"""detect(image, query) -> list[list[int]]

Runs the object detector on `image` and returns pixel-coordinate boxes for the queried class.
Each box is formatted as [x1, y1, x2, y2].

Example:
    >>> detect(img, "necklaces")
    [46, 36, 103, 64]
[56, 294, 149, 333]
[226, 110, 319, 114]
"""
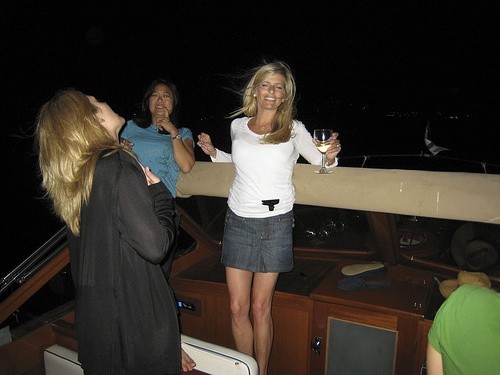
[252, 120, 273, 131]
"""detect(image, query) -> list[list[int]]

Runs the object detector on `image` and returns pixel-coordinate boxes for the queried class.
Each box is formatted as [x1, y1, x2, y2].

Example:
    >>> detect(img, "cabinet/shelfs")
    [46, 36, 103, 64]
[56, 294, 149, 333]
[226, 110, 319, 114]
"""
[168, 246, 449, 375]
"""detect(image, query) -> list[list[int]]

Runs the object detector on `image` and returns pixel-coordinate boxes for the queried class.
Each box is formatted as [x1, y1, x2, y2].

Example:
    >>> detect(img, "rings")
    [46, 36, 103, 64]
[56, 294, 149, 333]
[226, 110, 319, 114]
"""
[336, 145, 339, 147]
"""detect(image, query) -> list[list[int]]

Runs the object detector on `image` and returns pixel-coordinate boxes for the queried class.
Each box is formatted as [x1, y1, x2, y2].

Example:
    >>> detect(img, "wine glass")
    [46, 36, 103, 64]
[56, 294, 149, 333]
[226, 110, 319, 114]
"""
[314, 129, 333, 175]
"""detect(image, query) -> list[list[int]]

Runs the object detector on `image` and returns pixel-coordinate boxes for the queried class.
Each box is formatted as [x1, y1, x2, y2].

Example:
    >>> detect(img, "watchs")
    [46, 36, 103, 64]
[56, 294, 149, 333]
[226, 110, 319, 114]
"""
[172, 135, 181, 140]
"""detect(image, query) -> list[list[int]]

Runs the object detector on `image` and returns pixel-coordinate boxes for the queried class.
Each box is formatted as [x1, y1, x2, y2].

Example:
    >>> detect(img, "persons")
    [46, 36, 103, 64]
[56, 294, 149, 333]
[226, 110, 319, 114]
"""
[36, 90, 182, 375]
[426, 284, 500, 375]
[120, 79, 196, 372]
[197, 63, 341, 375]
